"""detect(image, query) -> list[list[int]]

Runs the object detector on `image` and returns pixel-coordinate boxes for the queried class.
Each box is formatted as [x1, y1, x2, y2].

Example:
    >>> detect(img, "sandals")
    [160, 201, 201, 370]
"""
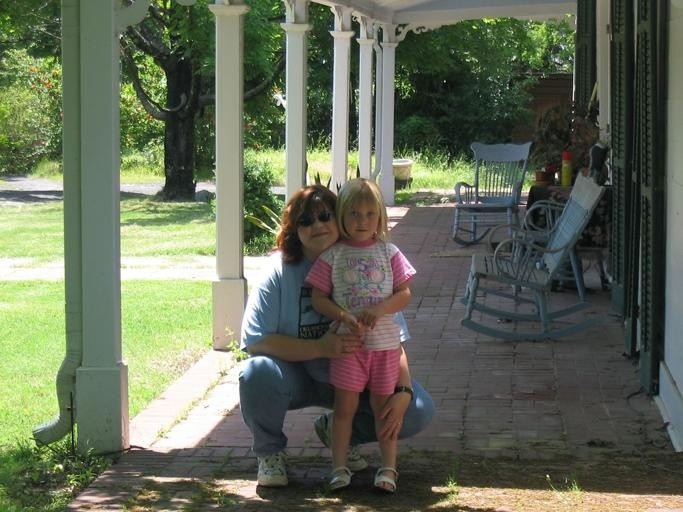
[374, 467, 399, 493]
[329, 466, 354, 490]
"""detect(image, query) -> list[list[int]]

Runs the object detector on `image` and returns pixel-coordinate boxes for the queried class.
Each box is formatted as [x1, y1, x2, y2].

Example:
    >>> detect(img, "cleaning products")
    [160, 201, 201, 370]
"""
[561, 151, 573, 186]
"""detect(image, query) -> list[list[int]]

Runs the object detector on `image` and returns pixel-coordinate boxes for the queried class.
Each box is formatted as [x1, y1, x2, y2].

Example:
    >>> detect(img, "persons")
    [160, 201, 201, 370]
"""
[306, 178, 417, 492]
[238, 184, 434, 487]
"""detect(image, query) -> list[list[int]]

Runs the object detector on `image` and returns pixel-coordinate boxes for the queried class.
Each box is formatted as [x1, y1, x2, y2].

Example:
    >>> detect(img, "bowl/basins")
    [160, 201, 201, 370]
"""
[393, 158, 413, 180]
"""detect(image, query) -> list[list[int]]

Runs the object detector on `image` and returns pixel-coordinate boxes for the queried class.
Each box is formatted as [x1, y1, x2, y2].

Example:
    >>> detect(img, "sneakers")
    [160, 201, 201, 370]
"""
[314, 414, 368, 471]
[257, 451, 288, 486]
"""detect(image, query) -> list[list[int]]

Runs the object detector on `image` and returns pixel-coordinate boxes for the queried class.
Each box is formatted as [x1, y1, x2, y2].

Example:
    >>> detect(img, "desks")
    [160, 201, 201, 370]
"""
[526, 186, 613, 226]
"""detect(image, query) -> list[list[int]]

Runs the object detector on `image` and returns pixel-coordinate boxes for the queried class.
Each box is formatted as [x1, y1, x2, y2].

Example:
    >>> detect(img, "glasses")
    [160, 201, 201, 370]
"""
[300, 210, 333, 227]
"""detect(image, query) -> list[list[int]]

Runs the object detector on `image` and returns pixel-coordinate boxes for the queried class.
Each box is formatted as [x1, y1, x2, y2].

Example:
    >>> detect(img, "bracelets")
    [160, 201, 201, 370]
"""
[395, 386, 414, 400]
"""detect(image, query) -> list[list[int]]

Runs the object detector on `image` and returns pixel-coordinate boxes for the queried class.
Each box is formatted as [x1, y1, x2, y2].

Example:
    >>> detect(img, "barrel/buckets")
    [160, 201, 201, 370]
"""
[392, 159, 413, 178]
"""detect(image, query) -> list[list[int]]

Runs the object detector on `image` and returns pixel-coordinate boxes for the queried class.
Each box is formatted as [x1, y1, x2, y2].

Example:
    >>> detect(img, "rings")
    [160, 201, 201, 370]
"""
[398, 422, 402, 424]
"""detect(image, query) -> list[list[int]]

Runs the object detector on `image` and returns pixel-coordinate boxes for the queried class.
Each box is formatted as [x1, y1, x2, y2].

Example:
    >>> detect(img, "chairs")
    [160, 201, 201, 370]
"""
[451, 137, 533, 251]
[461, 168, 605, 341]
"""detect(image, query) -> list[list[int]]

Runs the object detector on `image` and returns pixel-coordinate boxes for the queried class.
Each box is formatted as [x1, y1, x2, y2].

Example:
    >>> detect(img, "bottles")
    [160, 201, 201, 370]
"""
[561, 151, 572, 186]
